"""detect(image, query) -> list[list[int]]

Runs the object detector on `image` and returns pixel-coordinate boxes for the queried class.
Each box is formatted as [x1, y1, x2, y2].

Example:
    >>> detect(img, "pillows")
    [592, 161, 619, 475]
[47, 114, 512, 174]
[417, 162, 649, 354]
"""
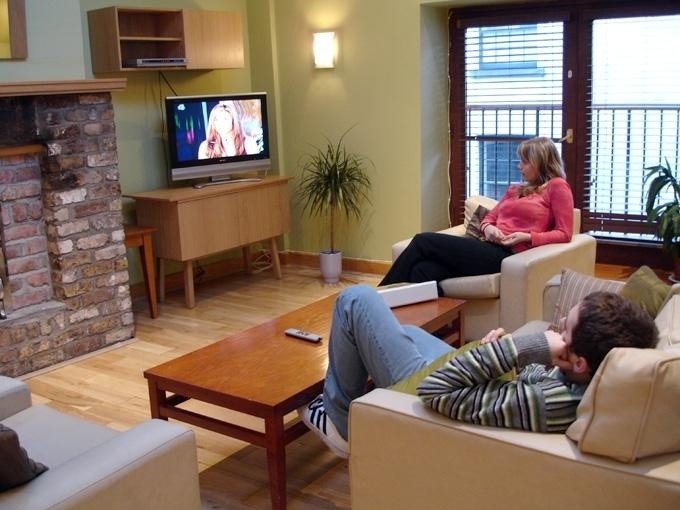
[461, 203, 497, 242]
[620, 264, 669, 320]
[0, 421, 50, 496]
[547, 264, 627, 332]
[385, 335, 516, 396]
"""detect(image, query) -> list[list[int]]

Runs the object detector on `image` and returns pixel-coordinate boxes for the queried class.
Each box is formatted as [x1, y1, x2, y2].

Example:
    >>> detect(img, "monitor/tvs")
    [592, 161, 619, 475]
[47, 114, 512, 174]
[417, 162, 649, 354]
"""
[165, 91, 271, 189]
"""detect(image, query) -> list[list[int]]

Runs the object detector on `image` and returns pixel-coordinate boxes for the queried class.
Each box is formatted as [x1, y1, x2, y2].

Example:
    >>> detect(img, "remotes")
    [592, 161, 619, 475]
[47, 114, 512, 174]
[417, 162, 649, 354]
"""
[285, 328, 322, 342]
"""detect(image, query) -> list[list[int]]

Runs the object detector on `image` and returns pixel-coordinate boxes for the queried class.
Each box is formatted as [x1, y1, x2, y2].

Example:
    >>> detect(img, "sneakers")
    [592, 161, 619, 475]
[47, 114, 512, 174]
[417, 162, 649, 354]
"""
[297, 393, 349, 460]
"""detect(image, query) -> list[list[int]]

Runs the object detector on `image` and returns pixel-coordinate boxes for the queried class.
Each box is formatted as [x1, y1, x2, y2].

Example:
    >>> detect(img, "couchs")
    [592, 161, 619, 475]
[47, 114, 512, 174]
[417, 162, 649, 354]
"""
[345, 267, 680, 509]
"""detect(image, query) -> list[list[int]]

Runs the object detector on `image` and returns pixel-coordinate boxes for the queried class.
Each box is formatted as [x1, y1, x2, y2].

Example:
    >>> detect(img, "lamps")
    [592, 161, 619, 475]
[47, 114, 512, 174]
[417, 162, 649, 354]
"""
[312, 31, 338, 69]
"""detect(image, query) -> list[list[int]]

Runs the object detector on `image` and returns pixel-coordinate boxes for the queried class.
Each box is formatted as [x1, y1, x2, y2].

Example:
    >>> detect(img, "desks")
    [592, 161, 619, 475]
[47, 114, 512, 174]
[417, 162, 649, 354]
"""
[122, 174, 299, 307]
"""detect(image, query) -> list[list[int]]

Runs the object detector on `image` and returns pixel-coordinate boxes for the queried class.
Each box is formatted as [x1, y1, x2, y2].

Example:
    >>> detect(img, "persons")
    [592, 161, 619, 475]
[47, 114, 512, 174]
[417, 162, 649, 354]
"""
[198, 103, 260, 160]
[296, 284, 661, 460]
[377, 136, 575, 296]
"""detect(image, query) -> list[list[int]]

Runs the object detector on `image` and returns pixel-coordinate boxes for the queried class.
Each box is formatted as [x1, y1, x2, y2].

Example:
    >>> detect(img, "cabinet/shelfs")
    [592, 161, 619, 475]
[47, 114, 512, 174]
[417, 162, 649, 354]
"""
[88, 6, 245, 75]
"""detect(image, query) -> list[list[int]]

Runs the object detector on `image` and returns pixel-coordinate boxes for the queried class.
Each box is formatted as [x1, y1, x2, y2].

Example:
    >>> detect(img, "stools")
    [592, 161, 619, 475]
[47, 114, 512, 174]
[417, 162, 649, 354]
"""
[123, 223, 162, 319]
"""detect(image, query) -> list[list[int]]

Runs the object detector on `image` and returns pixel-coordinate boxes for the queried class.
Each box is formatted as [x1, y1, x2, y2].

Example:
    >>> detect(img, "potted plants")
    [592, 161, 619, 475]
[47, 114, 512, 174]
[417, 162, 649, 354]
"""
[295, 124, 371, 280]
[639, 155, 680, 284]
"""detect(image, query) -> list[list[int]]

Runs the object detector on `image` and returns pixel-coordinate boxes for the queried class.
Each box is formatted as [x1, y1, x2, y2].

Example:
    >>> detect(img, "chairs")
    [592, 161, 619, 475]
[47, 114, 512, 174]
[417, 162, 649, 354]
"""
[0, 361, 202, 507]
[391, 191, 598, 337]
